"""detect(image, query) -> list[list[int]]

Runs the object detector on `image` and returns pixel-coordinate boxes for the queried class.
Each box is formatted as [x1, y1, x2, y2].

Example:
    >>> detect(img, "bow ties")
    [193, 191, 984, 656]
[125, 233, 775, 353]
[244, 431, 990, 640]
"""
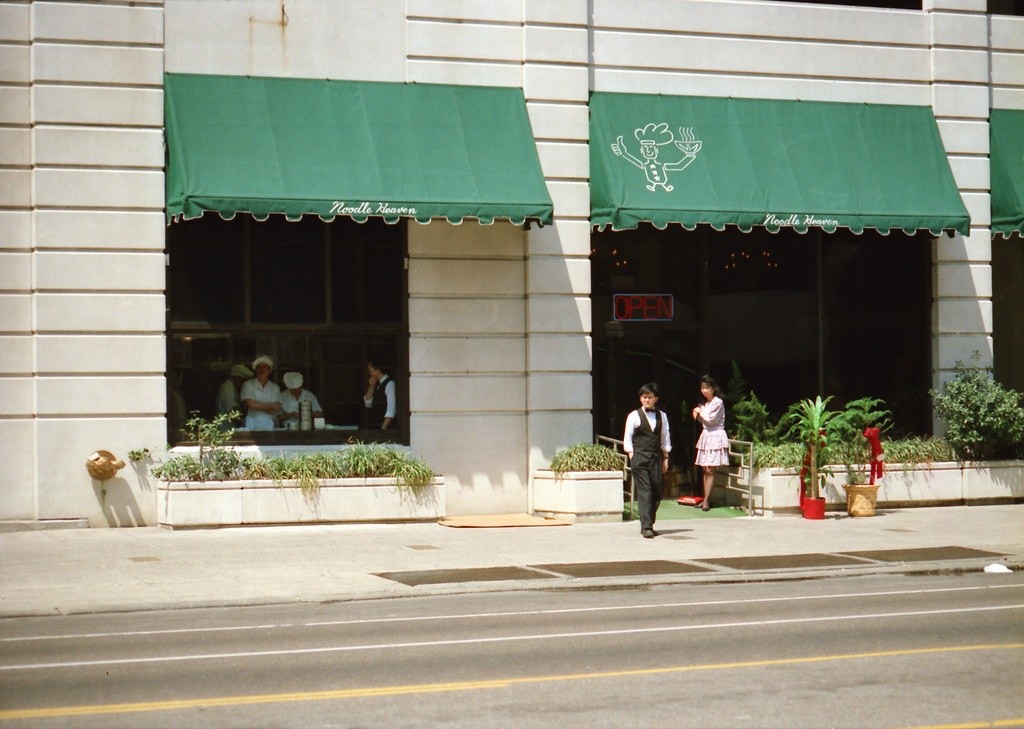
[645, 408, 655, 412]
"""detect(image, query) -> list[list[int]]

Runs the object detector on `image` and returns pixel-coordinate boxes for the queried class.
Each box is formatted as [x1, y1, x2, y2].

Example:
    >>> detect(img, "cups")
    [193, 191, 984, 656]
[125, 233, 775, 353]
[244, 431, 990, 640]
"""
[314, 418, 324, 429]
[288, 423, 298, 430]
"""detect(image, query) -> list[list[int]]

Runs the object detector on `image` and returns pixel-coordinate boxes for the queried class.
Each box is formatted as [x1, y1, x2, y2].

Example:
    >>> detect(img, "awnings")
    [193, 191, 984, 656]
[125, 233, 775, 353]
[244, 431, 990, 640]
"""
[588, 90, 971, 239]
[988, 108, 1024, 239]
[164, 71, 553, 230]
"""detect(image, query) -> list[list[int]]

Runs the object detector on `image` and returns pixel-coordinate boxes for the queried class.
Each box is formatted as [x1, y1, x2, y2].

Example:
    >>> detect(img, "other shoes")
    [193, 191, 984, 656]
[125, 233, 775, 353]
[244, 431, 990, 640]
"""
[702, 504, 710, 511]
[640, 528, 654, 538]
[694, 502, 711, 507]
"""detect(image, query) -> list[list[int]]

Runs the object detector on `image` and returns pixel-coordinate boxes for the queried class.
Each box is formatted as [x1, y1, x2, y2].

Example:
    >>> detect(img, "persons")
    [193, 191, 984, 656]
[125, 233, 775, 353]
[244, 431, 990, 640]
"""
[172, 391, 187, 437]
[624, 382, 673, 538]
[217, 364, 255, 429]
[240, 355, 282, 431]
[363, 359, 396, 431]
[277, 371, 323, 426]
[692, 375, 730, 511]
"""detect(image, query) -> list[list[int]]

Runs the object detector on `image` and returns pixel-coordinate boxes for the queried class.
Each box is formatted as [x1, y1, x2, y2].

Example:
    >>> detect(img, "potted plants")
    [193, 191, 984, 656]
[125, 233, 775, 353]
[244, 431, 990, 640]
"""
[737, 351, 1024, 520]
[128, 410, 446, 527]
[533, 441, 624, 513]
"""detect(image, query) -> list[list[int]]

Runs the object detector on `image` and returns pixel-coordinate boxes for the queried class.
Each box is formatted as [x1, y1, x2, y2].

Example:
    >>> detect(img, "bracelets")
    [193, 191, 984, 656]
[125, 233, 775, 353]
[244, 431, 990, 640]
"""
[663, 456, 669, 460]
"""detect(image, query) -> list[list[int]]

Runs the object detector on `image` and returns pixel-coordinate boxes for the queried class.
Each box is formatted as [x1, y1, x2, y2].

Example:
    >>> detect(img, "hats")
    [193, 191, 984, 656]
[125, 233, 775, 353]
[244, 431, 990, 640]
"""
[283, 372, 304, 389]
[229, 364, 254, 378]
[252, 355, 273, 370]
